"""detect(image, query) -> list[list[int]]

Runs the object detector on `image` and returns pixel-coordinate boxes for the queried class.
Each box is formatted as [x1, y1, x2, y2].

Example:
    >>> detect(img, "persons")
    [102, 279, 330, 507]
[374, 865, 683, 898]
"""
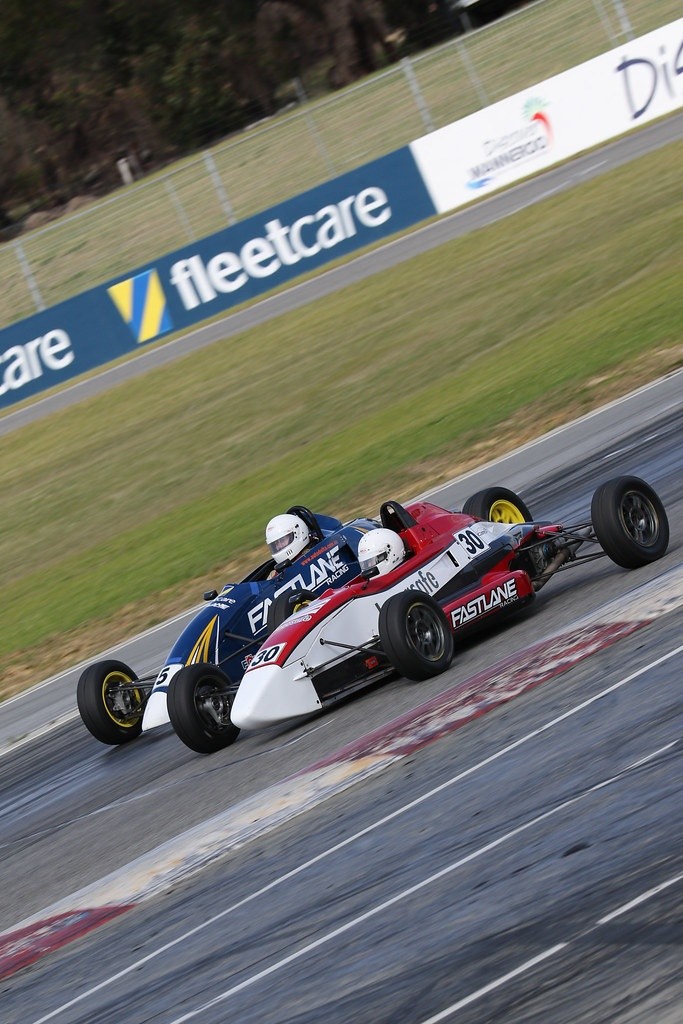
[355, 528, 410, 582]
[259, 514, 319, 582]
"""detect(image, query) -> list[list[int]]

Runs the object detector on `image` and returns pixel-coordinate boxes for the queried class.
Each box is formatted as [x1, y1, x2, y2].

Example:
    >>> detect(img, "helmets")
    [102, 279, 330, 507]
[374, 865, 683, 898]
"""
[357, 528, 406, 579]
[266, 514, 311, 565]
